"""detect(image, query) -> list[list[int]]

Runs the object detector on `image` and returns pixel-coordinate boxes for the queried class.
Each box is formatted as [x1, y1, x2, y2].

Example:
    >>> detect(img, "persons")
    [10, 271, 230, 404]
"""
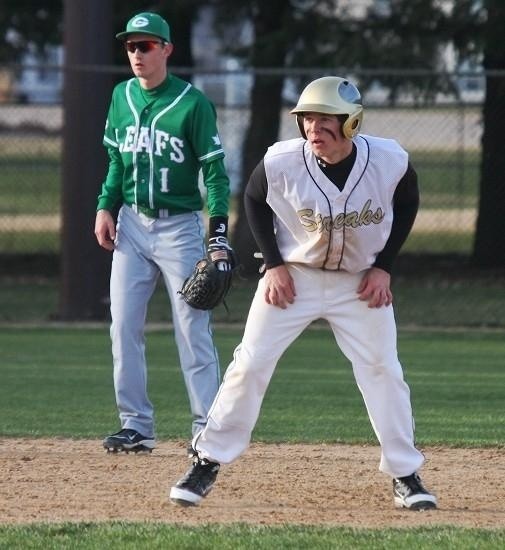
[94, 13, 241, 457]
[168, 77, 443, 514]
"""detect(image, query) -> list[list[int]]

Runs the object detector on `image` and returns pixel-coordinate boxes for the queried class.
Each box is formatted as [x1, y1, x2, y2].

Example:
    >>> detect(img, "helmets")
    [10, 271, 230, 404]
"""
[289, 76, 363, 141]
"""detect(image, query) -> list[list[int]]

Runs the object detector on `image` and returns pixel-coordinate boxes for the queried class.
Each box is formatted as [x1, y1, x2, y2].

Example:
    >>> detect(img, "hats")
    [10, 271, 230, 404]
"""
[115, 12, 171, 43]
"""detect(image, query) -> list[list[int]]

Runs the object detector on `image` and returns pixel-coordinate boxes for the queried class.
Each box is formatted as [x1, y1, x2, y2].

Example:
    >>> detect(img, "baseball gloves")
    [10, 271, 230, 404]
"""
[182, 259, 232, 310]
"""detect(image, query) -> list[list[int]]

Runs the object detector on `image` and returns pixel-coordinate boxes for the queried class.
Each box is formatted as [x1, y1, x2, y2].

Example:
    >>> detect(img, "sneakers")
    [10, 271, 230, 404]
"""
[170, 444, 220, 508]
[392, 472, 437, 509]
[102, 427, 156, 454]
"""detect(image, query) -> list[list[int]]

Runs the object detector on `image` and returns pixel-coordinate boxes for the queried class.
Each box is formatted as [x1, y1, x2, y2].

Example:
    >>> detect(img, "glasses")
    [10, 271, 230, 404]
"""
[123, 41, 159, 53]
